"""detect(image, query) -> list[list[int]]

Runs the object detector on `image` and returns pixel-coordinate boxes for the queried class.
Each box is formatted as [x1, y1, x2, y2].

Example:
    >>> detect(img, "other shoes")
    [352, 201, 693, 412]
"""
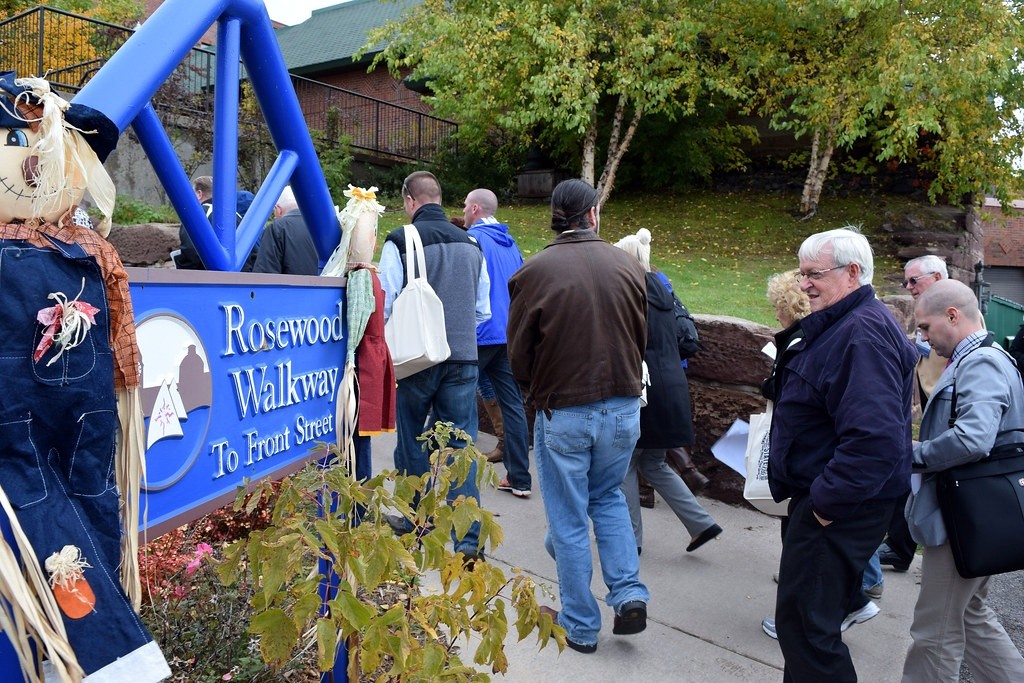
[773, 573, 779, 582]
[388, 515, 426, 535]
[865, 582, 883, 598]
[686, 523, 723, 552]
[687, 472, 709, 495]
[638, 488, 654, 508]
[481, 448, 504, 463]
[461, 550, 484, 571]
[637, 547, 641, 555]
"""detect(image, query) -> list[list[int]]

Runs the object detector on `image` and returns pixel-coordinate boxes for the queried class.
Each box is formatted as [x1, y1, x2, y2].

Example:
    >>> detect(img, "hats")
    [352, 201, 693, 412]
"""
[614, 228, 651, 271]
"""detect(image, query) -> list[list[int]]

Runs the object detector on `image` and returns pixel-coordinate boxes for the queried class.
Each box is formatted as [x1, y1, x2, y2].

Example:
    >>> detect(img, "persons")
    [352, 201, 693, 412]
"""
[377, 171, 492, 574]
[507, 178, 650, 653]
[463, 188, 534, 497]
[448, 215, 507, 463]
[767, 225, 920, 683]
[170, 176, 317, 275]
[756, 256, 1024, 638]
[316, 183, 396, 436]
[606, 228, 722, 557]
[0, 75, 173, 683]
[902, 278, 1024, 683]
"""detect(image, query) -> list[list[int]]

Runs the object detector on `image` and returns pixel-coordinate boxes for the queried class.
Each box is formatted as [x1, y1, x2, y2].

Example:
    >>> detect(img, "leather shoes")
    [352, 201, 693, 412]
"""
[613, 601, 647, 634]
[536, 606, 597, 653]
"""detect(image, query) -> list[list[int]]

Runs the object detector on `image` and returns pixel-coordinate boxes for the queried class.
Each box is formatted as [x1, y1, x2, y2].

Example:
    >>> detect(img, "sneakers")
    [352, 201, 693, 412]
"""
[491, 478, 531, 496]
[762, 617, 779, 638]
[841, 600, 881, 632]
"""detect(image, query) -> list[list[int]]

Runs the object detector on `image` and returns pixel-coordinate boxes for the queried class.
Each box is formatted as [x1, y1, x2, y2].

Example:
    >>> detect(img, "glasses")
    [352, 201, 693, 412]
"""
[795, 265, 863, 283]
[902, 272, 938, 288]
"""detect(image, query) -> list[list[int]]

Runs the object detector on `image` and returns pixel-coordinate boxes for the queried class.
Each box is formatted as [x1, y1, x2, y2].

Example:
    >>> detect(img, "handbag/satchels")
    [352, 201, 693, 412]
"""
[384, 225, 453, 379]
[744, 396, 792, 515]
[641, 360, 652, 407]
[936, 349, 1024, 578]
[674, 296, 701, 360]
